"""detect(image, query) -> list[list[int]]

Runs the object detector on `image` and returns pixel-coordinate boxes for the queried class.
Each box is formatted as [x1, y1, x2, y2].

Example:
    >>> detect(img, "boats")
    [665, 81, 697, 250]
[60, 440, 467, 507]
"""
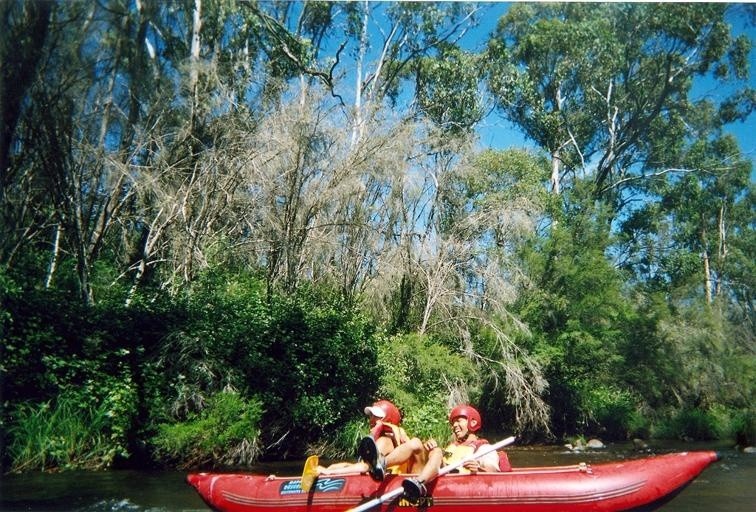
[179, 449, 720, 512]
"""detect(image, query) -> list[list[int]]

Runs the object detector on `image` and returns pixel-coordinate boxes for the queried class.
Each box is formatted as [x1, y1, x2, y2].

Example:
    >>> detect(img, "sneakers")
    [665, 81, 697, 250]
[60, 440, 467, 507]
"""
[400, 477, 428, 505]
[357, 434, 386, 483]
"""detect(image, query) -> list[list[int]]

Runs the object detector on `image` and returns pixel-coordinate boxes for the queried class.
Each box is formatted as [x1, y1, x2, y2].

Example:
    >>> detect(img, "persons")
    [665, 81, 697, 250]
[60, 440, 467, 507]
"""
[358, 403, 512, 509]
[313, 398, 417, 476]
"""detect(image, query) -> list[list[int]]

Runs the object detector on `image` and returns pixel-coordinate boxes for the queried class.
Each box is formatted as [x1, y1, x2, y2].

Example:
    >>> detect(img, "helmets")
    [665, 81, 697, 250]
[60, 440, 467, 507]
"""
[449, 405, 482, 431]
[363, 399, 402, 427]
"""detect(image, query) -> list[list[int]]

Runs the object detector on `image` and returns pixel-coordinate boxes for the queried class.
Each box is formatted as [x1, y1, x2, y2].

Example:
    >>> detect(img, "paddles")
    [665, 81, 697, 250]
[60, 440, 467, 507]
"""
[299, 455, 364, 494]
